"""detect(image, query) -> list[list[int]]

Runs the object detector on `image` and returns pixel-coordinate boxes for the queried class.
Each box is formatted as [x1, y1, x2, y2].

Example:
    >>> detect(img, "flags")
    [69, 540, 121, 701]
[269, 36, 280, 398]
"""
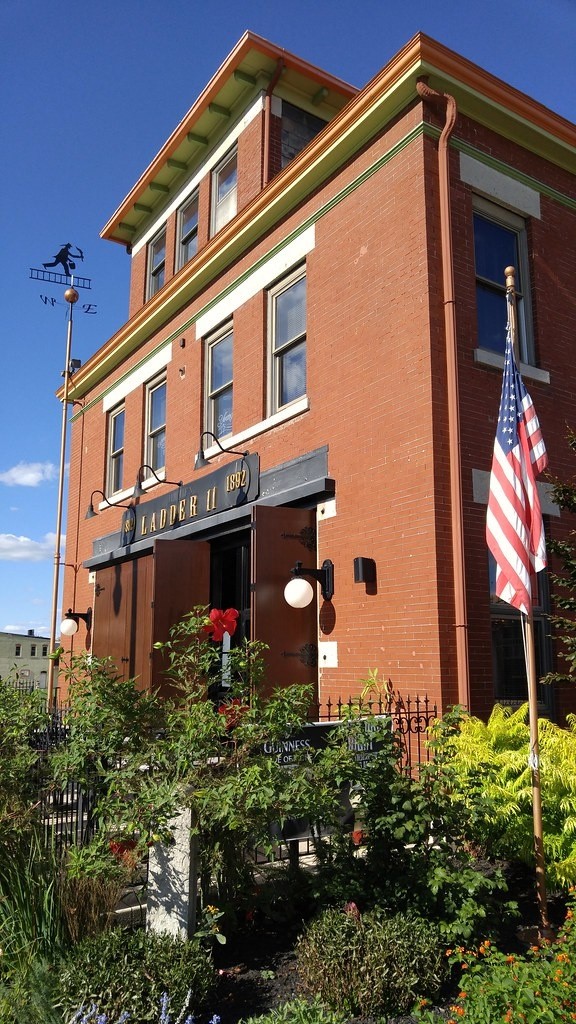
[485, 295, 551, 617]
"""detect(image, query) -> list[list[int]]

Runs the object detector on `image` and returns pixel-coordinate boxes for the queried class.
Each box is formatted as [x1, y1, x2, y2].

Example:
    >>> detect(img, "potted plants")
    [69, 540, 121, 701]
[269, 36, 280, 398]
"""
[41, 650, 175, 867]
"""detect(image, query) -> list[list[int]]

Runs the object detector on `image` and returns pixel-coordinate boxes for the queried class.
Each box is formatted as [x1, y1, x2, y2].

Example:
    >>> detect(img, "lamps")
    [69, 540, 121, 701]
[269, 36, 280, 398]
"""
[132, 465, 182, 497]
[84, 490, 131, 520]
[60, 607, 93, 635]
[193, 431, 250, 470]
[282, 559, 334, 608]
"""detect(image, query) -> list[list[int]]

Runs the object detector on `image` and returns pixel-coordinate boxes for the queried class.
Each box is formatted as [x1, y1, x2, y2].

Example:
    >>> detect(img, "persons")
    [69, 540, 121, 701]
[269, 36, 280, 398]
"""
[42, 242, 81, 277]
